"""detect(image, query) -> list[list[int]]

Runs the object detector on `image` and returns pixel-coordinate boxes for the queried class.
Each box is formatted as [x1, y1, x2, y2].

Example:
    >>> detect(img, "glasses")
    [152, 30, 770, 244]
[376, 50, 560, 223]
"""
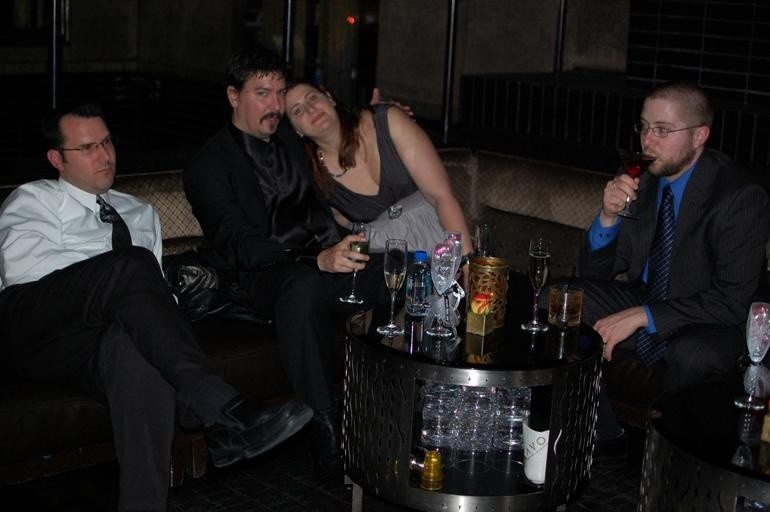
[63, 136, 119, 154]
[631, 122, 707, 139]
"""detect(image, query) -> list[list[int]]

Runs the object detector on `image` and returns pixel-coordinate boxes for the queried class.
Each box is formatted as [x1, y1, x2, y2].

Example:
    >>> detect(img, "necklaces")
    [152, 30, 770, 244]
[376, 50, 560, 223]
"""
[316, 144, 354, 178]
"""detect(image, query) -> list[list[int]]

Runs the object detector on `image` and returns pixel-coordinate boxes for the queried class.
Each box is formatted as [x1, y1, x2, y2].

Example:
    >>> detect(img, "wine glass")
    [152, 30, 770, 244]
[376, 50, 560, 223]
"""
[377, 238, 407, 336]
[520, 236, 551, 332]
[731, 405, 756, 469]
[341, 221, 372, 305]
[472, 222, 498, 255]
[424, 229, 464, 336]
[732, 303, 770, 411]
[613, 147, 655, 220]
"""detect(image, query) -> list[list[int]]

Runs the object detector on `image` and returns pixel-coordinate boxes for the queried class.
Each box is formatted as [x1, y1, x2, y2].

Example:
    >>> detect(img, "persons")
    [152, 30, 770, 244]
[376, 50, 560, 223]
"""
[527, 79, 765, 472]
[281, 79, 474, 257]
[0, 102, 316, 511]
[193, 48, 423, 479]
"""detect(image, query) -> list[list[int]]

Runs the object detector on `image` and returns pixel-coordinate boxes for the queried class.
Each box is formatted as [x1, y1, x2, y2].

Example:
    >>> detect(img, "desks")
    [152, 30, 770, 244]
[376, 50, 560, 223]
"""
[337, 296, 604, 509]
[636, 379, 770, 512]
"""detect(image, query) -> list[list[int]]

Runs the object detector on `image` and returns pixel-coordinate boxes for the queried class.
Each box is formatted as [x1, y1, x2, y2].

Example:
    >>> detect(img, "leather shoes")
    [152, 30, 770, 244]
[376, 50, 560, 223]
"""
[203, 393, 315, 467]
[596, 427, 629, 468]
[318, 408, 341, 465]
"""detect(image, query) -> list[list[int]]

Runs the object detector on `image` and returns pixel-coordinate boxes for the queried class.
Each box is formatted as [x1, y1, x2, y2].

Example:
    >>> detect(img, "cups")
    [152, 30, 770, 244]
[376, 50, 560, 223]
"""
[419, 450, 443, 490]
[549, 283, 584, 328]
[547, 325, 581, 360]
[422, 382, 529, 451]
[444, 455, 522, 476]
[520, 418, 551, 485]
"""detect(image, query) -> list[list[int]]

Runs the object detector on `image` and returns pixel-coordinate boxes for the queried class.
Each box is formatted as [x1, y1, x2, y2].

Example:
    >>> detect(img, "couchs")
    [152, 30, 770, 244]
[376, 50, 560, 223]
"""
[0, 146, 477, 503]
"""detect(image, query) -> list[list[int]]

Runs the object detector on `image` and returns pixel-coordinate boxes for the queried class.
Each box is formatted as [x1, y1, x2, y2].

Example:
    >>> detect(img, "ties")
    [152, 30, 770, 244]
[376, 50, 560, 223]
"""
[96, 194, 132, 251]
[635, 186, 675, 372]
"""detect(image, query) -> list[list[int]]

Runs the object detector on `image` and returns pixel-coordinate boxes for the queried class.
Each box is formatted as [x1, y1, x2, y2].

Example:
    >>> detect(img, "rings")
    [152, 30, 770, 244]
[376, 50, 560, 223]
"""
[610, 179, 617, 188]
[601, 340, 609, 346]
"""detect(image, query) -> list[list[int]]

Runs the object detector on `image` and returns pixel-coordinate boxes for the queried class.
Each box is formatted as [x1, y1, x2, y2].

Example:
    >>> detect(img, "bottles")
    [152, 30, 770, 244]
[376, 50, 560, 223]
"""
[406, 250, 432, 314]
[405, 315, 426, 353]
